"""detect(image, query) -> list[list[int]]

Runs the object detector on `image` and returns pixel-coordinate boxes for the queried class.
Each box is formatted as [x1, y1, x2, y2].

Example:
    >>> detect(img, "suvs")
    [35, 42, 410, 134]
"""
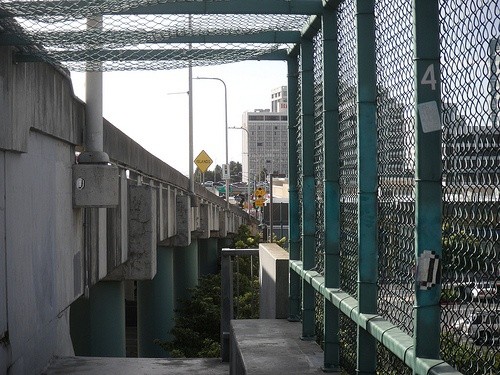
[462, 313, 500, 345]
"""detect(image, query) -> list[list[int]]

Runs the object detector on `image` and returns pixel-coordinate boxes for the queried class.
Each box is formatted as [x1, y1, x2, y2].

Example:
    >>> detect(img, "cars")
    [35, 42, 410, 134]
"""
[453, 313, 474, 330]
[468, 284, 496, 301]
[200, 177, 269, 194]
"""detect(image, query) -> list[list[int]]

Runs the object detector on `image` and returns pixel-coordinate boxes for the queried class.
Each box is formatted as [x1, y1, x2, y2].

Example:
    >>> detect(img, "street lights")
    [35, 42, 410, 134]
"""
[192, 77, 229, 207]
[226, 125, 250, 220]
